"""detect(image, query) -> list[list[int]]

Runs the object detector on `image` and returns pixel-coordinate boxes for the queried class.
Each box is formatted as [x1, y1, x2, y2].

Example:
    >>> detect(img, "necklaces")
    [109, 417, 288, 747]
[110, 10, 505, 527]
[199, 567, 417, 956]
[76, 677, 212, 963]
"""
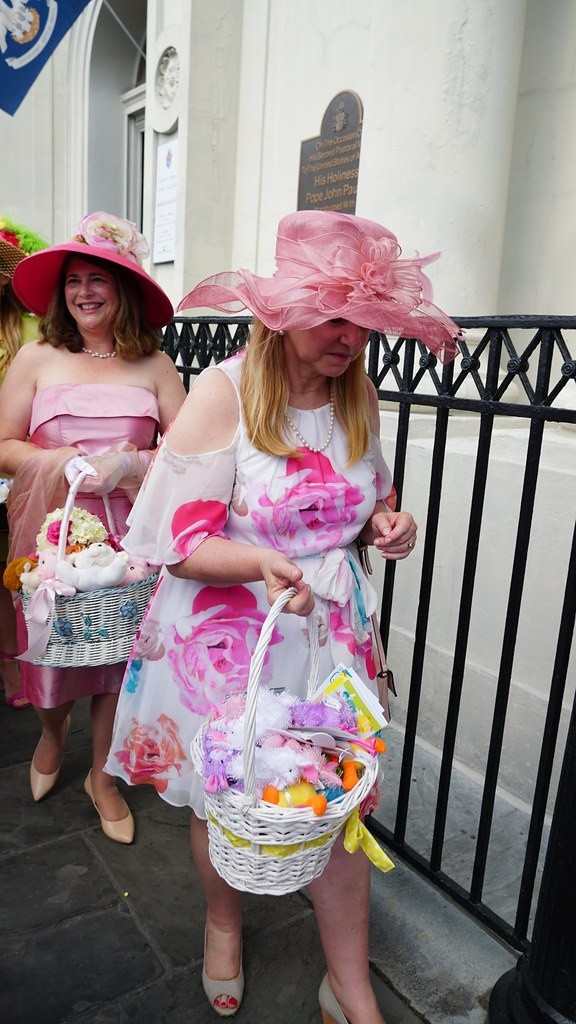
[286, 383, 335, 453]
[83, 347, 116, 358]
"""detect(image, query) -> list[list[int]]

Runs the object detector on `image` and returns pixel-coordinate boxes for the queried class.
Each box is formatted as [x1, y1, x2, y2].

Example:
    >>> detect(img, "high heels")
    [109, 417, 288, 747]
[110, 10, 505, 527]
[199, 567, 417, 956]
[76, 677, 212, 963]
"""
[29, 714, 71, 801]
[83, 768, 134, 844]
[319, 974, 350, 1024]
[201, 922, 244, 1016]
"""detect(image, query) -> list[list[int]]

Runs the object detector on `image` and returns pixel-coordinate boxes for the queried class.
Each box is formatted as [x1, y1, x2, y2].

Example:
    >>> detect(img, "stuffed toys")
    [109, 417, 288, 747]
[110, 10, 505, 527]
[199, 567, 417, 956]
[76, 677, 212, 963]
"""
[203, 689, 385, 814]
[3, 535, 161, 595]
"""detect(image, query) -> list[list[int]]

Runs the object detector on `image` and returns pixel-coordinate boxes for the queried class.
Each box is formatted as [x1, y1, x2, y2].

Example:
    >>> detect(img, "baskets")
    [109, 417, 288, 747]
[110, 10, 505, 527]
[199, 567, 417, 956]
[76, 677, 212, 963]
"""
[18, 474, 160, 667]
[191, 586, 379, 896]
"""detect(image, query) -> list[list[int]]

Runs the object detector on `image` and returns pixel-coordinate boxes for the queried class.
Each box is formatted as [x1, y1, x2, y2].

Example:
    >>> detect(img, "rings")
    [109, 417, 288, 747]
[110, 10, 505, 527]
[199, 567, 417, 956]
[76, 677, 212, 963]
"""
[406, 541, 412, 548]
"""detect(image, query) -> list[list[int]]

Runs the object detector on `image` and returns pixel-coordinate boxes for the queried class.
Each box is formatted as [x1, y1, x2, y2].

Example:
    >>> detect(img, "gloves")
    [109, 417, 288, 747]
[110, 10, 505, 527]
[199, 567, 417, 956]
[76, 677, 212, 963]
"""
[65, 454, 130, 496]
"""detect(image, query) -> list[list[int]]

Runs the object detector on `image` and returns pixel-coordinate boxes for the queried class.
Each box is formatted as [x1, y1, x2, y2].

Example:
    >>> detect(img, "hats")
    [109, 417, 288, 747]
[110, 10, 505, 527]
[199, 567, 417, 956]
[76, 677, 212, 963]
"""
[13, 212, 174, 332]
[0, 216, 48, 279]
[177, 211, 465, 365]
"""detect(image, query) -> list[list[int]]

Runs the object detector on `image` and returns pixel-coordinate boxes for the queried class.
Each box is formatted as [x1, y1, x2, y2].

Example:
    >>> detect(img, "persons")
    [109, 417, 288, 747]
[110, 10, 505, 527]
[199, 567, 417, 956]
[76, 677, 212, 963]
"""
[105, 211, 463, 1024]
[0, 219, 51, 707]
[0, 210, 190, 841]
